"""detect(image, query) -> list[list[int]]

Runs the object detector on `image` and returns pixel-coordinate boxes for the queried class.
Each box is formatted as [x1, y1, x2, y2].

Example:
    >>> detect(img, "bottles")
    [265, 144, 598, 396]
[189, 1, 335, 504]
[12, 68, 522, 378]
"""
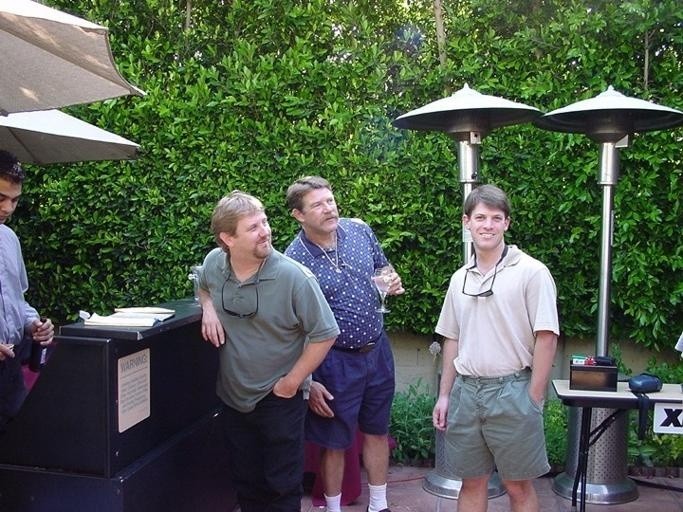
[28, 316, 47, 373]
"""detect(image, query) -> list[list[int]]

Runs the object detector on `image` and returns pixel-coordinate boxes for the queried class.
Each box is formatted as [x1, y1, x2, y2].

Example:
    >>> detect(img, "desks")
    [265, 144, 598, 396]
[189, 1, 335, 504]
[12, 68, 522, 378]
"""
[552, 378, 683, 512]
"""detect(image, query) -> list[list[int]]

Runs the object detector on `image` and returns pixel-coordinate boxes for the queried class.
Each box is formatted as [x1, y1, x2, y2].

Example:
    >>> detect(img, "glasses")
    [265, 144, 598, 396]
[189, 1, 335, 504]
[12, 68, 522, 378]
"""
[221, 279, 259, 318]
[461, 265, 498, 297]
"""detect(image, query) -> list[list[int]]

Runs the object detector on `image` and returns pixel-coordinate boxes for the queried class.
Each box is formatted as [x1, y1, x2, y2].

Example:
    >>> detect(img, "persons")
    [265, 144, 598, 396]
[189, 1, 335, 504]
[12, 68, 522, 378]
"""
[431, 184, 560, 512]
[0, 149, 55, 428]
[281, 175, 406, 512]
[197, 189, 341, 511]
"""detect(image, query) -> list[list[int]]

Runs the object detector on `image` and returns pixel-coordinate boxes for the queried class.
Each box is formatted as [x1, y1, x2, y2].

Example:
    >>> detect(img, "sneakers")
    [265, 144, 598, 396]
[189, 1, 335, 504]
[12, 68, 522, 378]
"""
[366, 504, 394, 512]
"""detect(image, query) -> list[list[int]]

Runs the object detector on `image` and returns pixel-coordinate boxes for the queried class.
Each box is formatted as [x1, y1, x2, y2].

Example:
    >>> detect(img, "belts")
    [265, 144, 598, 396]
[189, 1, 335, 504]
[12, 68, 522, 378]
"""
[332, 329, 384, 353]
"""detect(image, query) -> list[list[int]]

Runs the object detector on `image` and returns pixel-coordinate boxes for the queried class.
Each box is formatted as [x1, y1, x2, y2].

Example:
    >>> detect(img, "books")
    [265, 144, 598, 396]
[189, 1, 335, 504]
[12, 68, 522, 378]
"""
[83, 306, 176, 328]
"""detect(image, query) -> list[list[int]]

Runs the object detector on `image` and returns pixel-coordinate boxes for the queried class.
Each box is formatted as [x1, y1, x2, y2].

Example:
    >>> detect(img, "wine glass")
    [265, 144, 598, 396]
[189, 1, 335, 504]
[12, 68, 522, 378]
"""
[374, 268, 391, 314]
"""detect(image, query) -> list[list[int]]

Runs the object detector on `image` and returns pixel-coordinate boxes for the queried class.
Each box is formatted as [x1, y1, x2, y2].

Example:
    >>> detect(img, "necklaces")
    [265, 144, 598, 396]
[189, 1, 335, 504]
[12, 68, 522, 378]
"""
[306, 229, 341, 274]
